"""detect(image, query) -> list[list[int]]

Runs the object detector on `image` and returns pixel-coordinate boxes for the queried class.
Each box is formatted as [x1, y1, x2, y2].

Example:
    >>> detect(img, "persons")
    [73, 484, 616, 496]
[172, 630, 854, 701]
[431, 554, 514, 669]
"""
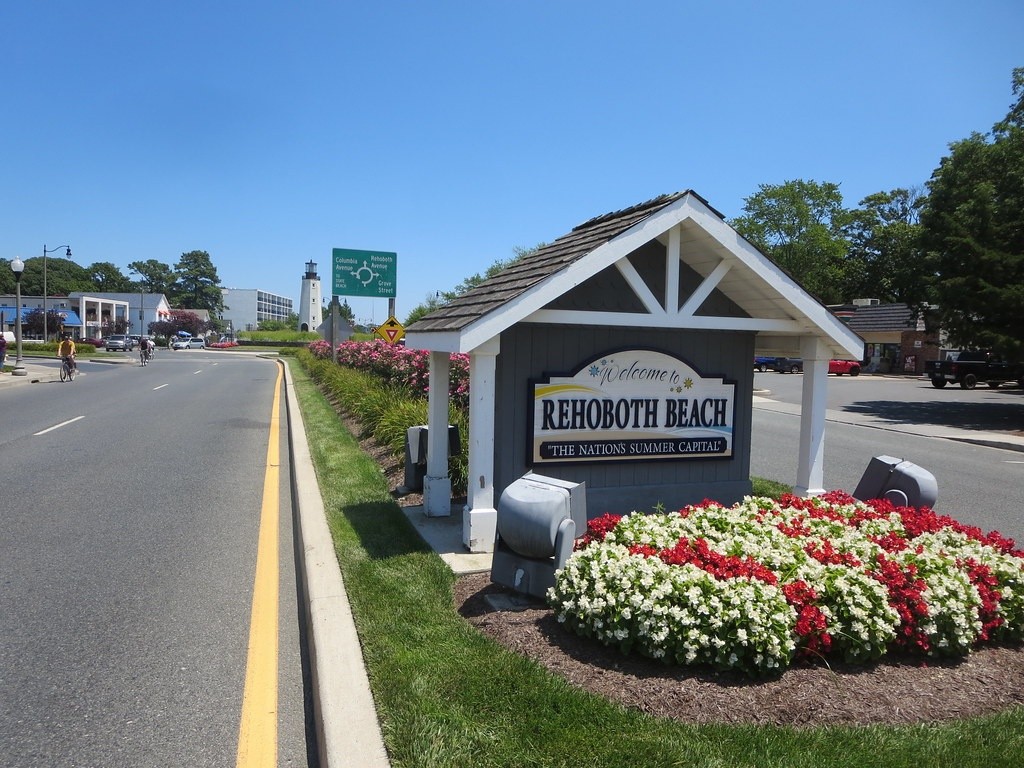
[138, 337, 156, 365]
[57, 336, 76, 379]
[0, 333, 7, 370]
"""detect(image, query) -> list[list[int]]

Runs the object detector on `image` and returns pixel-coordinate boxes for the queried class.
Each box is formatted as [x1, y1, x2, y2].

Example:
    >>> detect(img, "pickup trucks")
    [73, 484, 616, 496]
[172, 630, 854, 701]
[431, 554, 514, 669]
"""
[924, 349, 1024, 390]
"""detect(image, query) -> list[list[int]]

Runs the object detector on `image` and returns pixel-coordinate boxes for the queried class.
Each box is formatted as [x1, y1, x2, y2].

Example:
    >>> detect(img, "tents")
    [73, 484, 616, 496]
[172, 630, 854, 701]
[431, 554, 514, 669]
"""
[176, 331, 192, 338]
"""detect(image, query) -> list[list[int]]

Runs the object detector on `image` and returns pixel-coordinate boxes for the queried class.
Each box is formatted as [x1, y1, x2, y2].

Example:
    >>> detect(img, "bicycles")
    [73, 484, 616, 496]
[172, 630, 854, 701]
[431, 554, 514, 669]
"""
[58, 355, 76, 382]
[140, 349, 153, 366]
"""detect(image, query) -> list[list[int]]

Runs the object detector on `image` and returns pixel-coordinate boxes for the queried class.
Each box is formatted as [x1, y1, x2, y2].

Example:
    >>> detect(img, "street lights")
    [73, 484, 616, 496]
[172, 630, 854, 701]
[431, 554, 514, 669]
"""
[10, 256, 26, 368]
[44, 245, 72, 343]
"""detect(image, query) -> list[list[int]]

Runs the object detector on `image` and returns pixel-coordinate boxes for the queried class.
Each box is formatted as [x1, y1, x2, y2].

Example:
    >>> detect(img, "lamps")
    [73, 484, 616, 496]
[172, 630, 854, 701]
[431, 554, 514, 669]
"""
[852, 455, 938, 509]
[489, 470, 587, 599]
[404, 424, 460, 490]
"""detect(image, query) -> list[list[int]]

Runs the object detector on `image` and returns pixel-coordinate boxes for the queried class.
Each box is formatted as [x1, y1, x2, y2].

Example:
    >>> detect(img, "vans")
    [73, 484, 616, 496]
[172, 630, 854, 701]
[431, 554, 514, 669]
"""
[172, 338, 205, 350]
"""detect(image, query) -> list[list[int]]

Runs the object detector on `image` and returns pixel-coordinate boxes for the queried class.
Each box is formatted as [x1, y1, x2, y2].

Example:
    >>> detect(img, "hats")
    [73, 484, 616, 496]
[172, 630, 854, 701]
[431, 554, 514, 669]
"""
[64, 336, 68, 339]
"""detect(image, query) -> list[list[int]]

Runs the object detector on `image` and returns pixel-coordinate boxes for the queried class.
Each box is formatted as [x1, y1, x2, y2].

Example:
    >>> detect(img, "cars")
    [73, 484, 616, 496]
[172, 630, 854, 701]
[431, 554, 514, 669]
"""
[754, 357, 803, 374]
[73, 333, 151, 348]
[828, 360, 862, 376]
[105, 334, 133, 352]
